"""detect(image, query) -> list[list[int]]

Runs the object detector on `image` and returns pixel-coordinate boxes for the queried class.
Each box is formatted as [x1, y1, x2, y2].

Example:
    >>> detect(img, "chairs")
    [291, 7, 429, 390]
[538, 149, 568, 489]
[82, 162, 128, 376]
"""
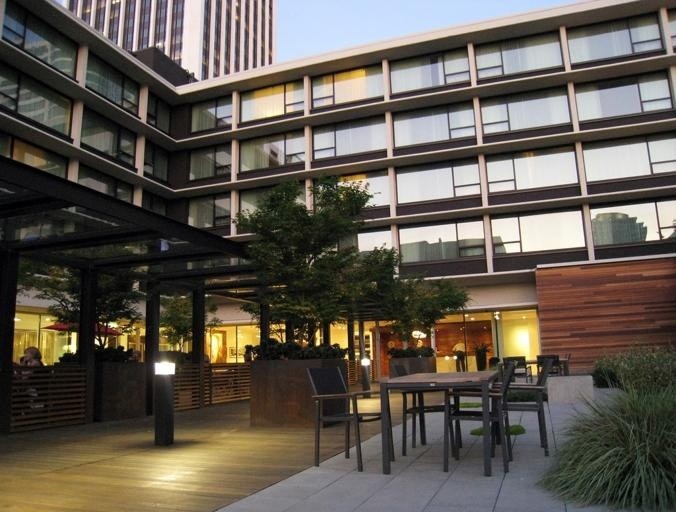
[559, 354, 567, 375]
[560, 353, 571, 375]
[535, 354, 562, 377]
[306, 358, 556, 473]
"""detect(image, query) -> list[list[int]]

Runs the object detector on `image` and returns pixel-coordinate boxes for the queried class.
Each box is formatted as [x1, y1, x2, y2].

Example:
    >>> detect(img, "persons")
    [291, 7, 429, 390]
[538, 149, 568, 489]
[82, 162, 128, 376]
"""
[14, 347, 46, 421]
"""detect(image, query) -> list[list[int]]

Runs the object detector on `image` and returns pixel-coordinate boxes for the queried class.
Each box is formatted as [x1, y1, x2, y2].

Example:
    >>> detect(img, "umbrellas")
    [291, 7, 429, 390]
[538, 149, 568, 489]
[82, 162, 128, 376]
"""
[39, 318, 123, 337]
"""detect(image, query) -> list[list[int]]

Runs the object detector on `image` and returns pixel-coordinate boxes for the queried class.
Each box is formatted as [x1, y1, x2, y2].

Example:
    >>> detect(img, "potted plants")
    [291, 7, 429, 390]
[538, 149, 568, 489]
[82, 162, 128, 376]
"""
[470, 342, 494, 371]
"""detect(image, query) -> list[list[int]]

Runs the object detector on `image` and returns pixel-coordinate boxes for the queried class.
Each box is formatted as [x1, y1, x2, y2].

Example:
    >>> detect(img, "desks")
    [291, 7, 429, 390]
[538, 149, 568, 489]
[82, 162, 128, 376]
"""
[379, 370, 499, 477]
[497, 358, 569, 377]
